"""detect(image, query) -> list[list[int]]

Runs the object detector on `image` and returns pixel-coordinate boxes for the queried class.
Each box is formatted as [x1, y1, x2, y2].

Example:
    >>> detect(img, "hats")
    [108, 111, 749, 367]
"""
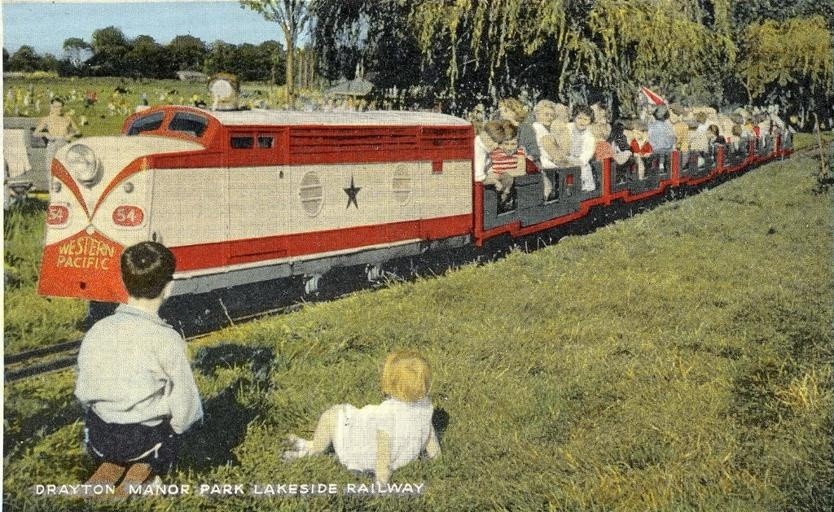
[669, 104, 684, 114]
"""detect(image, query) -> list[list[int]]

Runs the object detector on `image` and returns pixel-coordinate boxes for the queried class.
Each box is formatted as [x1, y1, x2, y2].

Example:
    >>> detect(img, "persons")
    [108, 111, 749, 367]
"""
[280, 350, 441, 495]
[73, 240, 205, 504]
[32, 97, 81, 195]
[3, 81, 376, 140]
[475, 98, 791, 211]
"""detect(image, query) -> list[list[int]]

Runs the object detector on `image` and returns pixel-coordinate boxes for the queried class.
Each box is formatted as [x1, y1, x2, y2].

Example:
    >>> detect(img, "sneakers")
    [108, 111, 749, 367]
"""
[105, 462, 159, 507]
[78, 460, 127, 496]
